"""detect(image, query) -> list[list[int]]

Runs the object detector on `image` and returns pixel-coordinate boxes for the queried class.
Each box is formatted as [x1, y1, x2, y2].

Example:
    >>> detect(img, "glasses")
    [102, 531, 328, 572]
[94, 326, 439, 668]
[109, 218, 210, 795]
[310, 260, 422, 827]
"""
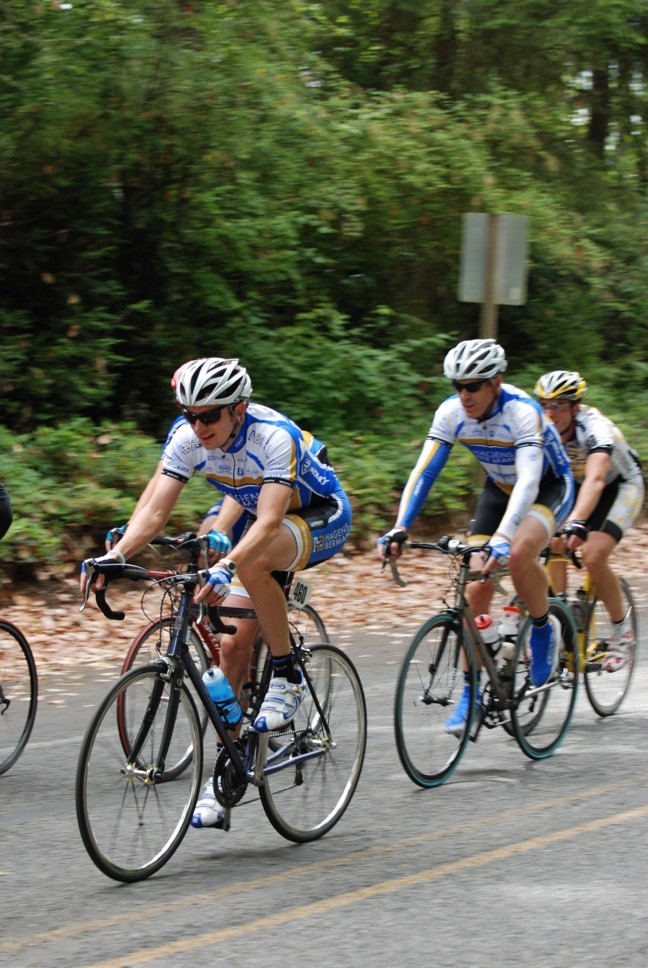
[181, 405, 228, 425]
[539, 402, 571, 409]
[452, 379, 489, 393]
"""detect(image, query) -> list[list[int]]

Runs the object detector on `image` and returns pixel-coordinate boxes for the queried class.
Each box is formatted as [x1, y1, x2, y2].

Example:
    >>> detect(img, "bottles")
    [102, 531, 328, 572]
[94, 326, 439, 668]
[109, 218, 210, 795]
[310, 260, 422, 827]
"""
[475, 613, 504, 660]
[556, 591, 568, 606]
[202, 668, 243, 723]
[208, 621, 222, 642]
[498, 606, 520, 660]
[573, 587, 588, 632]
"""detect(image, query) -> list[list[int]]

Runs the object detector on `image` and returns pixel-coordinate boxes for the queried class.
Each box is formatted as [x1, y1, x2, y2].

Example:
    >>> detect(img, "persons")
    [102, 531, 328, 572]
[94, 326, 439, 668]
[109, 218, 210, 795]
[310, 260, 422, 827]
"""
[534, 371, 647, 674]
[377, 339, 575, 733]
[80, 354, 351, 827]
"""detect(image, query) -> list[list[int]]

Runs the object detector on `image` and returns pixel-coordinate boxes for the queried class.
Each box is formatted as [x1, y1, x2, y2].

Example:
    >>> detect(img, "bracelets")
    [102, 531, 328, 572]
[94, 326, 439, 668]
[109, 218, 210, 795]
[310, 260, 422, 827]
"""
[106, 549, 125, 568]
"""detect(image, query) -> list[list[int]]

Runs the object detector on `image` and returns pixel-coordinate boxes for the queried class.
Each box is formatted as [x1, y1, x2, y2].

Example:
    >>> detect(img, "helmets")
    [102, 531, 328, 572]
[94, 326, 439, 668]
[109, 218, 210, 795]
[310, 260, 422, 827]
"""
[443, 338, 508, 380]
[533, 370, 588, 400]
[176, 357, 252, 407]
[171, 360, 192, 391]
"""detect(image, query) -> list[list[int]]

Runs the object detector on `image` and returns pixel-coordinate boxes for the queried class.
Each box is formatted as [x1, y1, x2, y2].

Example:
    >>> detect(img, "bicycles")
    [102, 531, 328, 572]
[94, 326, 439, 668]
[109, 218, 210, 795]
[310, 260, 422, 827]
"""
[74, 519, 369, 883]
[0, 617, 40, 777]
[379, 531, 639, 789]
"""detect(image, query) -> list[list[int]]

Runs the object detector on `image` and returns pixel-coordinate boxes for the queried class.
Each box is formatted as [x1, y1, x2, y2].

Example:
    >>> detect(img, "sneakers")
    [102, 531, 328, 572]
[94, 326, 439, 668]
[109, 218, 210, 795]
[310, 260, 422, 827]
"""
[254, 668, 308, 732]
[601, 605, 632, 673]
[530, 615, 561, 688]
[445, 684, 482, 732]
[191, 776, 226, 828]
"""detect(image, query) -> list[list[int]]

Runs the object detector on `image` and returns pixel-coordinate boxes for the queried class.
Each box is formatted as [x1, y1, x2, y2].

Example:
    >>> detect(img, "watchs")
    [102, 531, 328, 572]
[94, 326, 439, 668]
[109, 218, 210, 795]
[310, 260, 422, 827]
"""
[219, 558, 238, 575]
